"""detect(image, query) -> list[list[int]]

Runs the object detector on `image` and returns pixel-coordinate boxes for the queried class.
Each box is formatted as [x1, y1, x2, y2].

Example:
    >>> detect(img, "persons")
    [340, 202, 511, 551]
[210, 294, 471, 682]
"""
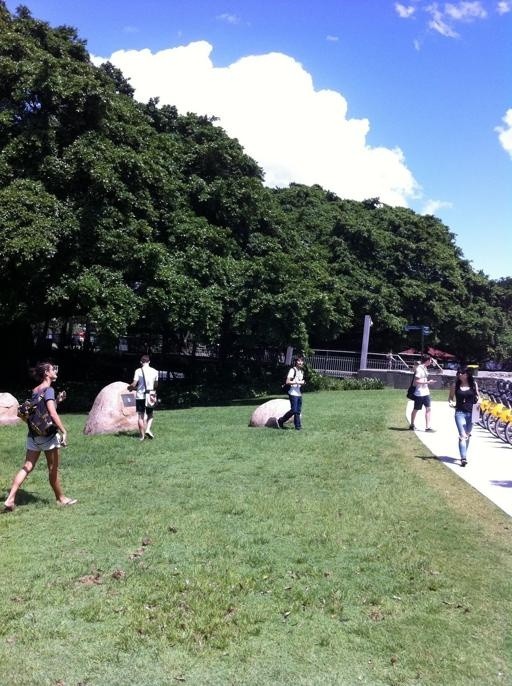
[4, 363, 76, 510]
[448, 365, 481, 466]
[386, 348, 394, 369]
[409, 353, 437, 431]
[276, 356, 306, 429]
[78, 328, 85, 347]
[127, 354, 159, 440]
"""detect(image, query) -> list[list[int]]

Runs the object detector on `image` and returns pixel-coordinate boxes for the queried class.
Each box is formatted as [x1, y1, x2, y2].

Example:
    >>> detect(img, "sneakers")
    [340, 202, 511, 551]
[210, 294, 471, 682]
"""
[295, 426, 302, 430]
[276, 418, 284, 429]
[409, 425, 418, 431]
[425, 428, 436, 433]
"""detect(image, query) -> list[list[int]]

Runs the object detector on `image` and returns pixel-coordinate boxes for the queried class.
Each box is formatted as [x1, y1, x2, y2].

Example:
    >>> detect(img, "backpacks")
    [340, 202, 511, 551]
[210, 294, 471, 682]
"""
[144, 388, 160, 410]
[17, 385, 59, 442]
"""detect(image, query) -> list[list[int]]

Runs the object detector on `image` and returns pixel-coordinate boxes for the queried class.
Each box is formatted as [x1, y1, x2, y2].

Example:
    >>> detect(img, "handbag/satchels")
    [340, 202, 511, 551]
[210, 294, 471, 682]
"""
[281, 378, 293, 393]
[471, 403, 481, 424]
[407, 385, 417, 401]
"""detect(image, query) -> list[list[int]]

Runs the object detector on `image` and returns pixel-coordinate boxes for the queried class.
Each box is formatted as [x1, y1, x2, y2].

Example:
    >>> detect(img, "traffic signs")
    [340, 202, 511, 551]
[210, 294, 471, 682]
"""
[423, 325, 430, 335]
[404, 324, 423, 331]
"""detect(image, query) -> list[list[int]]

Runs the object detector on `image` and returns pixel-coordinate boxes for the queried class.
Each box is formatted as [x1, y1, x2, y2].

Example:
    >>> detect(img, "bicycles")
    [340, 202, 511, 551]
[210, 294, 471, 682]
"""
[475, 372, 512, 446]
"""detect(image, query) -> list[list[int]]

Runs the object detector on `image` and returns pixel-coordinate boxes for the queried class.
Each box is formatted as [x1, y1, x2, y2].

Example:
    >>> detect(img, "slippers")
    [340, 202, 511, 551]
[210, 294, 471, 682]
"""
[56, 496, 78, 506]
